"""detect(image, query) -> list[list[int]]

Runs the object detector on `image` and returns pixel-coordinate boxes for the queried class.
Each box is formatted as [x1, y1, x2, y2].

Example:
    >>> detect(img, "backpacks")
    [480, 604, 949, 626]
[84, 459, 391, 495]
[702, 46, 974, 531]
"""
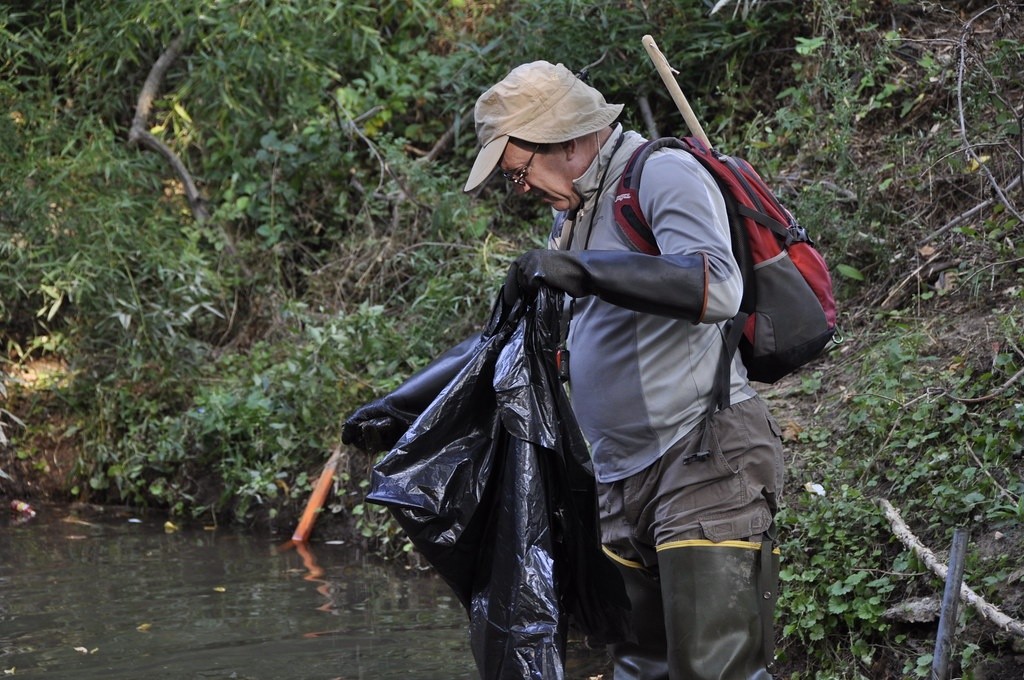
[612, 135, 837, 409]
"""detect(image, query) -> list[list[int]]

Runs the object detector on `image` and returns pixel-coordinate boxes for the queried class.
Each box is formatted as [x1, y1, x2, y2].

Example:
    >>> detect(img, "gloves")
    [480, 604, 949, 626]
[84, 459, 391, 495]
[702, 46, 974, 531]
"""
[342, 330, 485, 453]
[504, 248, 709, 326]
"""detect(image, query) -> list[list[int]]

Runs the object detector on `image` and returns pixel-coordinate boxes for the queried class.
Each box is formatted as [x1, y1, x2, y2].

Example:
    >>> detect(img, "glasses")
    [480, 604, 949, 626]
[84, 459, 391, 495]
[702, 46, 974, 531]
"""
[502, 144, 540, 185]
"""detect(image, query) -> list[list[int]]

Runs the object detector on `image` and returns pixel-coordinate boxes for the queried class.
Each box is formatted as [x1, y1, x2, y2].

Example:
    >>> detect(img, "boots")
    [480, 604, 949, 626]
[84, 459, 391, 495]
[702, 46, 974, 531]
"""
[599, 540, 781, 680]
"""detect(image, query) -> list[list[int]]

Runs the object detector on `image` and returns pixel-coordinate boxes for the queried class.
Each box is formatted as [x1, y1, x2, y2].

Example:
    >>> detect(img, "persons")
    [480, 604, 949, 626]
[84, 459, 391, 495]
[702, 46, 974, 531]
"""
[342, 60, 785, 680]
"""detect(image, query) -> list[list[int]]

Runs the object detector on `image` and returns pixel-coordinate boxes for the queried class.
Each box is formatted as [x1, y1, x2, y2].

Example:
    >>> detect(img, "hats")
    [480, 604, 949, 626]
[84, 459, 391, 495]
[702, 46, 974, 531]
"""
[464, 60, 625, 193]
